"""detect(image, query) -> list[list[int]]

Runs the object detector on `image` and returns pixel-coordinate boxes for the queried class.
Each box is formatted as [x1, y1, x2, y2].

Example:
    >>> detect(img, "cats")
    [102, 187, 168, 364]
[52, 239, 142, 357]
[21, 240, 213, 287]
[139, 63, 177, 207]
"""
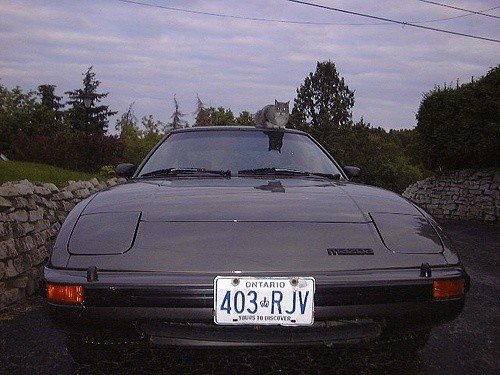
[254, 99, 291, 130]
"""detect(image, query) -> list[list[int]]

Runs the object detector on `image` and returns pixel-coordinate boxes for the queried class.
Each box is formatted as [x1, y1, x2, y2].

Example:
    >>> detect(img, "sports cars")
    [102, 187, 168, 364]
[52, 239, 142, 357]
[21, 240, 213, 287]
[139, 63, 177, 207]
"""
[38, 121, 472, 373]
